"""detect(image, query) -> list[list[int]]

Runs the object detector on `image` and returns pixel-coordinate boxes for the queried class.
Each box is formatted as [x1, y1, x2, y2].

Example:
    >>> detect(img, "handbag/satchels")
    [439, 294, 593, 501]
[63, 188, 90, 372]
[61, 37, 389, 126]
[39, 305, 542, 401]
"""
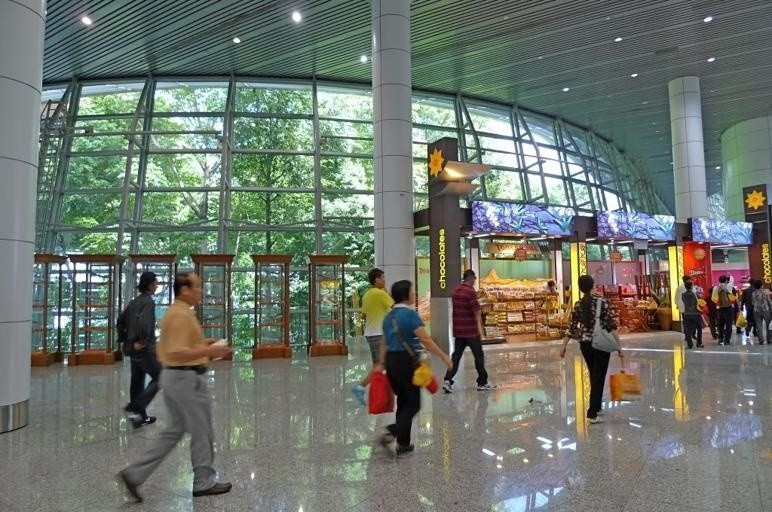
[367, 371, 395, 415]
[692, 292, 708, 315]
[388, 310, 438, 394]
[610, 352, 642, 402]
[592, 296, 619, 353]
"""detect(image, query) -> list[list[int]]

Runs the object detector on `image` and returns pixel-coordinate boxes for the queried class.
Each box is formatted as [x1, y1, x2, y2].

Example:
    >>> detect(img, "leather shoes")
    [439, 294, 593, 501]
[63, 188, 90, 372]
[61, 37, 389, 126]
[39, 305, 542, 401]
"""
[192, 481, 232, 498]
[115, 471, 142, 502]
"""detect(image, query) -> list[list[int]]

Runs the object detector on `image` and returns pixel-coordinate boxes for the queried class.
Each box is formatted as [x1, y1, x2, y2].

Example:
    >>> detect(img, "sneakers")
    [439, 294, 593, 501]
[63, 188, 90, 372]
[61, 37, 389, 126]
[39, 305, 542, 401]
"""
[441, 380, 454, 394]
[597, 409, 606, 415]
[351, 385, 366, 406]
[395, 443, 415, 455]
[138, 415, 157, 424]
[586, 417, 603, 424]
[475, 382, 497, 390]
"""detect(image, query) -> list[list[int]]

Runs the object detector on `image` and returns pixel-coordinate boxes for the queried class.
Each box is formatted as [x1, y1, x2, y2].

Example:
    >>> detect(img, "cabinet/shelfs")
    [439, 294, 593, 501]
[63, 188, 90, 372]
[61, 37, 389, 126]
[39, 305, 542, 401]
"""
[533, 292, 562, 342]
[478, 297, 536, 335]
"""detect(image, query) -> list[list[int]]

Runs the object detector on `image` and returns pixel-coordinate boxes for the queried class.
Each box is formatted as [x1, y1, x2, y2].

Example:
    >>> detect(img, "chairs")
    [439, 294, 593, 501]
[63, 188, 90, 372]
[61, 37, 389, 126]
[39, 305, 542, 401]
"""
[612, 300, 649, 332]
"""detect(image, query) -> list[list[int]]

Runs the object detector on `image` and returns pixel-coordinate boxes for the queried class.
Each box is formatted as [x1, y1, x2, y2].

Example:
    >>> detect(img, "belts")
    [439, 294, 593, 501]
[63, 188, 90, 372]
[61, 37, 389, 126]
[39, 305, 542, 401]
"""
[159, 363, 202, 371]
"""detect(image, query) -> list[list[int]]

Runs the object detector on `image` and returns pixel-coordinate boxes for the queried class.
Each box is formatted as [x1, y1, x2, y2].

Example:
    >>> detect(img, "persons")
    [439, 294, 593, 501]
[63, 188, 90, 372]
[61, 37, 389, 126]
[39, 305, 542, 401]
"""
[441, 270, 497, 393]
[741, 278, 756, 336]
[117, 272, 163, 429]
[359, 280, 454, 456]
[751, 280, 772, 345]
[348, 268, 397, 406]
[763, 311, 764, 312]
[715, 274, 740, 346]
[682, 280, 705, 349]
[703, 287, 722, 340]
[726, 272, 744, 334]
[538, 280, 557, 307]
[675, 275, 697, 341]
[559, 274, 625, 424]
[114, 270, 234, 504]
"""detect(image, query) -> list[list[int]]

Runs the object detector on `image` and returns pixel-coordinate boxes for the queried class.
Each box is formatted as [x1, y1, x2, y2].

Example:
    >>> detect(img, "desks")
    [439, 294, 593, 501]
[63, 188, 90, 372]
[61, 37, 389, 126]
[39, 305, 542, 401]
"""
[627, 306, 657, 332]
